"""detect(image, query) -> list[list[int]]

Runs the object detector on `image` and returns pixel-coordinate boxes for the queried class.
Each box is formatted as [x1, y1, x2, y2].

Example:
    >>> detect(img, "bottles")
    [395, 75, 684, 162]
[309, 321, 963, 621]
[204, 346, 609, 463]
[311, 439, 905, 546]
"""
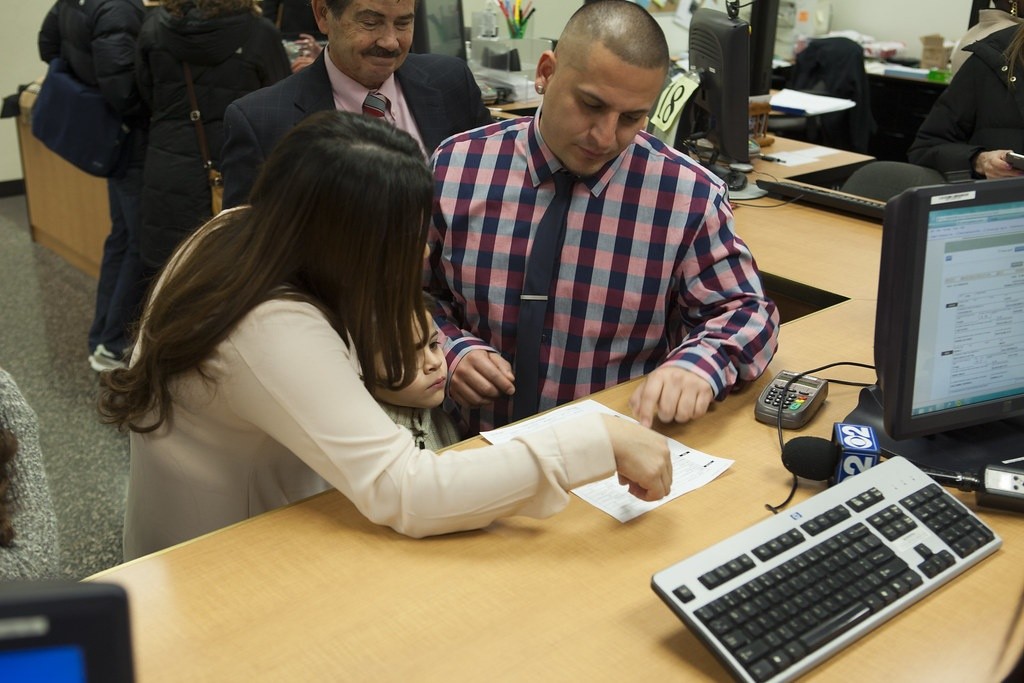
[794, 11, 813, 60]
[479, 1, 499, 38]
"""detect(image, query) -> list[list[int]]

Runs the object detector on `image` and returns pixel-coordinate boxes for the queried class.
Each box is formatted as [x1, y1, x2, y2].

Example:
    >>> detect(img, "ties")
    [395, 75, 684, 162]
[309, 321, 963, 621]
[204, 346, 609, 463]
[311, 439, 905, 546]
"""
[511, 173, 579, 421]
[362, 92, 388, 125]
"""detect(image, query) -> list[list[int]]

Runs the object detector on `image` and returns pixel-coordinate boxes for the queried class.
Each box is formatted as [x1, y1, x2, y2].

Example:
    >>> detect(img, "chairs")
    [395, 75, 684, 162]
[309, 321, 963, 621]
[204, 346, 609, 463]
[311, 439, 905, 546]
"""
[836, 161, 947, 203]
[769, 37, 874, 187]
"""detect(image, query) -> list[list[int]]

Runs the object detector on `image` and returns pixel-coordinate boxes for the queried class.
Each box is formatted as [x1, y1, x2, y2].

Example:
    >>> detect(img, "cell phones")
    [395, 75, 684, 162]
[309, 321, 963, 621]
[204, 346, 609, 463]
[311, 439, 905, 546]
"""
[1005, 151, 1024, 171]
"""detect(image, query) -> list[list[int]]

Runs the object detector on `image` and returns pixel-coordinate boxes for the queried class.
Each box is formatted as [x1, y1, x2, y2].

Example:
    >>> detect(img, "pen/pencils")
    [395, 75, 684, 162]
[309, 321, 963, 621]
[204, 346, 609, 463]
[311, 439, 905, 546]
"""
[498, 0, 536, 38]
[760, 154, 787, 163]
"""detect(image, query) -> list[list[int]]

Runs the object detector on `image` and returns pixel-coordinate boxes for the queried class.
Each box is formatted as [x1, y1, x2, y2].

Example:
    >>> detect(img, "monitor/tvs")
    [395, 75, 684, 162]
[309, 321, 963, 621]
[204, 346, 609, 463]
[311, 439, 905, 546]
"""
[839, 176, 1024, 477]
[411, 0, 469, 65]
[644, 0, 780, 190]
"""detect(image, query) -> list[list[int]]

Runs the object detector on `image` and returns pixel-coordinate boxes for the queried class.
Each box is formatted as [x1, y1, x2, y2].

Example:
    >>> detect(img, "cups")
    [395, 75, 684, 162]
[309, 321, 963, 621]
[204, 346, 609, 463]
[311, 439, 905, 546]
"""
[507, 17, 526, 38]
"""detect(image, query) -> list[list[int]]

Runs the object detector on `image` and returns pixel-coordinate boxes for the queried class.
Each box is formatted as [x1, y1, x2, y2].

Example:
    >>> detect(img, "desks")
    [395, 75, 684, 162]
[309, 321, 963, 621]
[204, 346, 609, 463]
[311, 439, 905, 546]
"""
[4, 50, 1024, 683]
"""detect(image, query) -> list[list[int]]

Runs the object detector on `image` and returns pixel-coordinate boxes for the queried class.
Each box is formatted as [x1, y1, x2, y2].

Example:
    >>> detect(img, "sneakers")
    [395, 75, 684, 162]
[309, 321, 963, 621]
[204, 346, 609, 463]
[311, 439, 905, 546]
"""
[88, 340, 129, 375]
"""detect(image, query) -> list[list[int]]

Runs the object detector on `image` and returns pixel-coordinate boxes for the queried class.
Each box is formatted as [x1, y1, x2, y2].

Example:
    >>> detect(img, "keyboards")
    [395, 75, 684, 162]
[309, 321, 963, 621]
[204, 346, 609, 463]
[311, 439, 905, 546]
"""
[652, 455, 1001, 683]
[754, 179, 885, 220]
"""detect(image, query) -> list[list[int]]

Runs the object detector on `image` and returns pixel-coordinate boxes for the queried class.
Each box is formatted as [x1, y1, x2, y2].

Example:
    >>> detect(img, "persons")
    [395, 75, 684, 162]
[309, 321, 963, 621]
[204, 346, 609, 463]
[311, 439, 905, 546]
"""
[371, 302, 461, 453]
[422, 0, 780, 443]
[95, 110, 673, 564]
[843, 21, 1024, 201]
[0, 369, 59, 578]
[37, 0, 494, 367]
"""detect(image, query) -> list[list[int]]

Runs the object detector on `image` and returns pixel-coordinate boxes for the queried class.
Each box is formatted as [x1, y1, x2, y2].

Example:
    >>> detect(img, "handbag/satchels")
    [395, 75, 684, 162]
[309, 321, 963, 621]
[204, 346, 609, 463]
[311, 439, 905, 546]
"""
[30, 60, 129, 178]
[207, 168, 225, 217]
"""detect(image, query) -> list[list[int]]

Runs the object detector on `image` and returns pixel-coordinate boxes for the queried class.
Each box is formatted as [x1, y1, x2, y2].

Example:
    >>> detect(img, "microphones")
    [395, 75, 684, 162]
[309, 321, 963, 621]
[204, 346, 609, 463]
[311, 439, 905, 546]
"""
[782, 422, 1024, 513]
